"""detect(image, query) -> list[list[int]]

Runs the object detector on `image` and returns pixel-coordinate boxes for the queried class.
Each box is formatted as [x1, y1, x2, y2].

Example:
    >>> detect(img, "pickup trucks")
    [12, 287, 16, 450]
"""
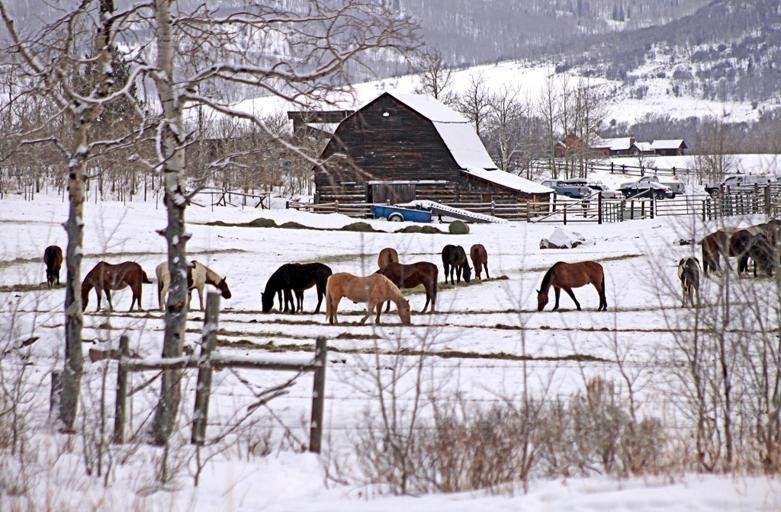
[703, 172, 777, 200]
[538, 175, 687, 200]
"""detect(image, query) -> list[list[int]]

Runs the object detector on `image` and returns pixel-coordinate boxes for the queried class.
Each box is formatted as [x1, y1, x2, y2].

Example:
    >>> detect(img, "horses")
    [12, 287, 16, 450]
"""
[535, 260, 607, 311]
[373, 248, 438, 313]
[81, 261, 152, 312]
[702, 219, 781, 278]
[441, 244, 490, 285]
[325, 272, 411, 325]
[156, 260, 231, 312]
[678, 256, 702, 308]
[44, 246, 63, 287]
[260, 263, 332, 313]
